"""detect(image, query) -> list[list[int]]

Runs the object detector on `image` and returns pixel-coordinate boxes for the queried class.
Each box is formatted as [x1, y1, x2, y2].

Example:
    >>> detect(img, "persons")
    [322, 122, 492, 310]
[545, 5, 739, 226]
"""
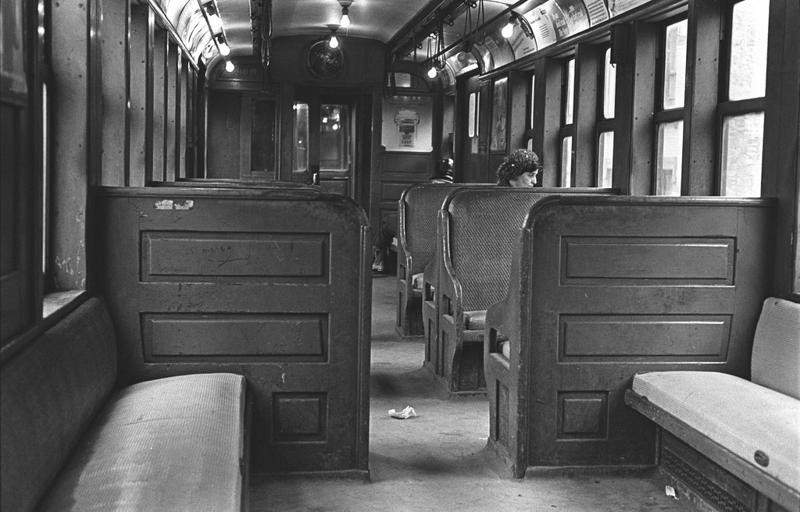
[495, 148, 541, 187]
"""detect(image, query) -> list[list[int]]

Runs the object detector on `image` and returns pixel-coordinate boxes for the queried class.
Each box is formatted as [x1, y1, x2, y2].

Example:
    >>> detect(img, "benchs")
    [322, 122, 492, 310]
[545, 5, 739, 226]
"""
[97, 175, 367, 478]
[629, 287, 800, 510]
[390, 176, 775, 473]
[2, 288, 247, 512]
